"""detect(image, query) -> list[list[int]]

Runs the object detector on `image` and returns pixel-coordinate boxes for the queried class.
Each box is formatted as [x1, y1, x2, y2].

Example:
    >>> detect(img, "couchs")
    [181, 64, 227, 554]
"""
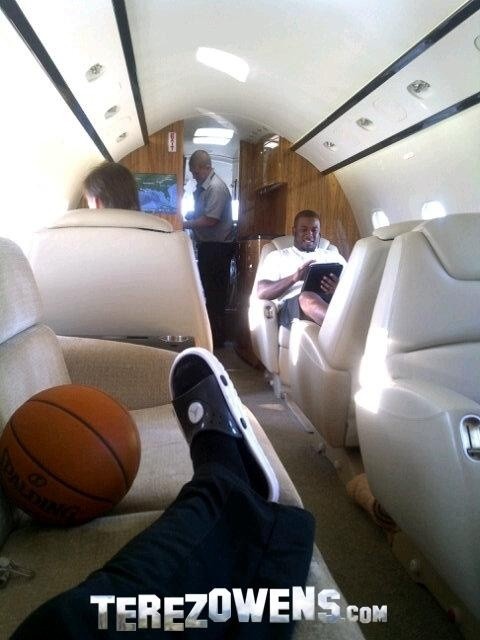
[0, 235, 365, 640]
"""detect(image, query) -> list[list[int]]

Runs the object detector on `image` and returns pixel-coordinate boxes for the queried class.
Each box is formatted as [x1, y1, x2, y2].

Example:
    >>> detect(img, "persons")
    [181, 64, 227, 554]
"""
[80, 162, 140, 210]
[183, 149, 235, 347]
[256, 209, 340, 330]
[8, 347, 316, 639]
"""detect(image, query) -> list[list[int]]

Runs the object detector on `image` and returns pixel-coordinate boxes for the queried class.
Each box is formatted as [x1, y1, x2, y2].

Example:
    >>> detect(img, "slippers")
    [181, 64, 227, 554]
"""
[168, 345, 280, 503]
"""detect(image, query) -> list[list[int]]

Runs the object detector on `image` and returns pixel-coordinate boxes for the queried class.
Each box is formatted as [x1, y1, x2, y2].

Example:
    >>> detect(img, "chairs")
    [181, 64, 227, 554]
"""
[289, 219, 428, 449]
[246, 221, 348, 388]
[20, 209, 213, 353]
[354, 211, 479, 640]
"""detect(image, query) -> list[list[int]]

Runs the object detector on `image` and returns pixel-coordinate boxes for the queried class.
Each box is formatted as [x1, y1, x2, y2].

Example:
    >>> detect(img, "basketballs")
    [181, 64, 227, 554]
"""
[0, 384, 141, 527]
